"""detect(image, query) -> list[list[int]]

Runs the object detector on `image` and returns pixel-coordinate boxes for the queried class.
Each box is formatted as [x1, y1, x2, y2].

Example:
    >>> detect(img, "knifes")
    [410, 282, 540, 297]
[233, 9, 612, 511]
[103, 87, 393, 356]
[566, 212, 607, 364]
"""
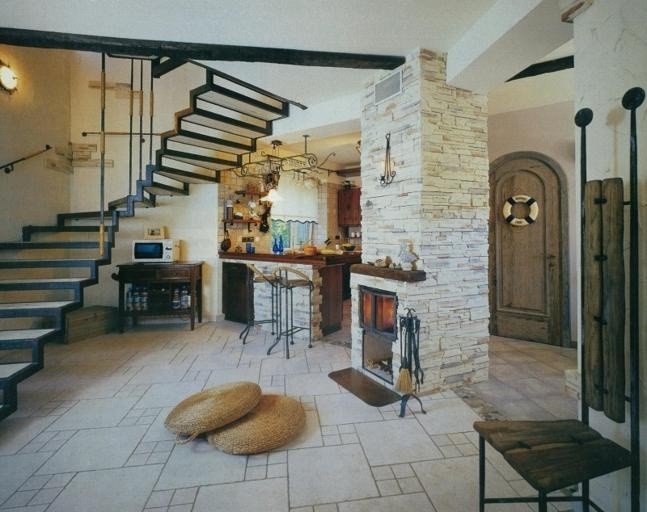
[324, 238, 331, 246]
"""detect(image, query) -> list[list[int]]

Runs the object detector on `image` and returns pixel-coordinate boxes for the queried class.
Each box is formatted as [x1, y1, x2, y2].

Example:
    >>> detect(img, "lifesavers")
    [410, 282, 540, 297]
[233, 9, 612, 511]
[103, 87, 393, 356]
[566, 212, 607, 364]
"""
[503, 195, 539, 226]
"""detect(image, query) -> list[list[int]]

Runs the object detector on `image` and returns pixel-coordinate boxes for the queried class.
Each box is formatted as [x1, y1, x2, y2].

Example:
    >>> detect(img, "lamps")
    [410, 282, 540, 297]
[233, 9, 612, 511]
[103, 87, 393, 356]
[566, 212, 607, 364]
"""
[0, 60, 18, 95]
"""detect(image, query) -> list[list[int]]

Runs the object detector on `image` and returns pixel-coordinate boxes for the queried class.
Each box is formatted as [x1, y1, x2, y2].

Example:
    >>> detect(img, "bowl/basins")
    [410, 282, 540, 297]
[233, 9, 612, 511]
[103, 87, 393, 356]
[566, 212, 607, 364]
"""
[345, 245, 355, 251]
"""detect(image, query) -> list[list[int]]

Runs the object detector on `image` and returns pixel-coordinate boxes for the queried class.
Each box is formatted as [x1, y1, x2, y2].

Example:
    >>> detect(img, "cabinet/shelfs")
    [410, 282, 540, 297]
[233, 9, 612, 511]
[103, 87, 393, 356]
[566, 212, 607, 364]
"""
[338, 188, 361, 225]
[112, 261, 204, 332]
[222, 190, 267, 234]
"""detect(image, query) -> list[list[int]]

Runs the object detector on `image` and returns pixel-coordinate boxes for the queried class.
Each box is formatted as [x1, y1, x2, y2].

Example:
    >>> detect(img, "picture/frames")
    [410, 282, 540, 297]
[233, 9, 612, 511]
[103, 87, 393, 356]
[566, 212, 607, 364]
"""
[144, 225, 164, 240]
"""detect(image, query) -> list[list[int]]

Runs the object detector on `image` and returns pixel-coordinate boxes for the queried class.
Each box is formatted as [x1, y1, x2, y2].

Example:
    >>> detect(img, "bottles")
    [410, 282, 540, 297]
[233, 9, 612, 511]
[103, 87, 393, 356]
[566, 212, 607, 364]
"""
[127, 286, 149, 312]
[172, 285, 188, 310]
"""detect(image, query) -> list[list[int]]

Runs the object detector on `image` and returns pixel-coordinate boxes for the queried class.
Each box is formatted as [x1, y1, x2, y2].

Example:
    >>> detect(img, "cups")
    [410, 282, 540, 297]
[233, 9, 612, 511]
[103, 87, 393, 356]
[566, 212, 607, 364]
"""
[351, 232, 361, 237]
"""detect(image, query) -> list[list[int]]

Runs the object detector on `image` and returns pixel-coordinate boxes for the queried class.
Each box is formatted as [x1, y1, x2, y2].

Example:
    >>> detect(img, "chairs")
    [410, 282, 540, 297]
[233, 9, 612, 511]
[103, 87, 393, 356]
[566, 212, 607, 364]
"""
[238, 262, 283, 345]
[266, 265, 314, 360]
[471, 84, 647, 512]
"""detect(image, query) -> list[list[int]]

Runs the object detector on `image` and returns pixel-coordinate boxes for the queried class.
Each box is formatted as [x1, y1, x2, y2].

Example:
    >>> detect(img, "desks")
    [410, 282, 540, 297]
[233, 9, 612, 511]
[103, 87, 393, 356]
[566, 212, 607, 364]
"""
[218, 251, 345, 337]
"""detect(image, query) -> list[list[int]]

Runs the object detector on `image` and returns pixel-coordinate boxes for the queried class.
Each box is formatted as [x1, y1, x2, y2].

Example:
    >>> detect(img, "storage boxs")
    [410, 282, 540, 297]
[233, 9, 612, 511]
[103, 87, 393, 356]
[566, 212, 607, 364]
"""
[42, 305, 118, 345]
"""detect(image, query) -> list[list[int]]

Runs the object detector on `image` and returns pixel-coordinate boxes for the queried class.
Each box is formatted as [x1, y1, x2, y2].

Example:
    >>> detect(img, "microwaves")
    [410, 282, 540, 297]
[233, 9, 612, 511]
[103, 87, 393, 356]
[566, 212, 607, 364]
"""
[132, 239, 180, 263]
